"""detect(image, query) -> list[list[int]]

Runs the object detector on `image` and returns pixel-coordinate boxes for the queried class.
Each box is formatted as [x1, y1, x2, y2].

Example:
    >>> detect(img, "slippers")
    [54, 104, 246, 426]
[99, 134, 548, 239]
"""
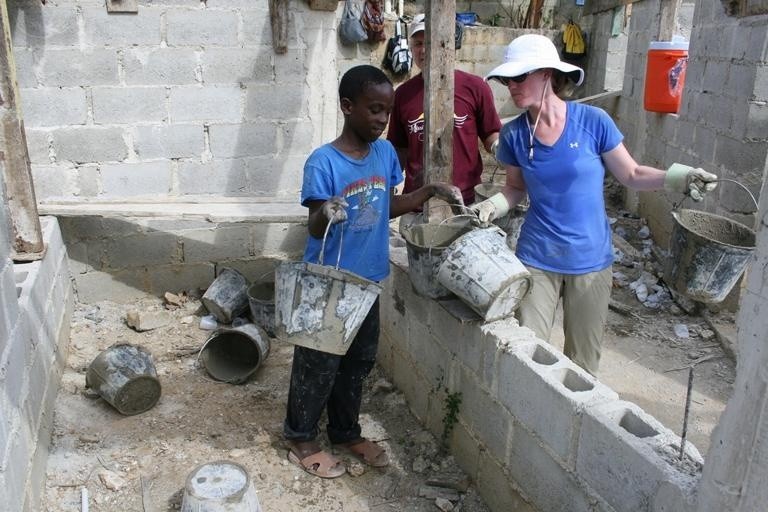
[287, 449, 345, 479]
[333, 439, 389, 467]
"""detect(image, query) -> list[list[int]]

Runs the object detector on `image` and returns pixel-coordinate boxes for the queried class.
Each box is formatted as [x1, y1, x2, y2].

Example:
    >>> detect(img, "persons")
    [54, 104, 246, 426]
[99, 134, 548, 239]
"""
[280, 63, 471, 478]
[462, 34, 719, 379]
[385, 12, 502, 235]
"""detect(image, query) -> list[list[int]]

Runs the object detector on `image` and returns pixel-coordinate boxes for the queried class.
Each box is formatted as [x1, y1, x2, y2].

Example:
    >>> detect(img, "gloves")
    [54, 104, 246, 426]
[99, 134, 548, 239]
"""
[490, 138, 507, 170]
[468, 192, 510, 228]
[664, 162, 718, 202]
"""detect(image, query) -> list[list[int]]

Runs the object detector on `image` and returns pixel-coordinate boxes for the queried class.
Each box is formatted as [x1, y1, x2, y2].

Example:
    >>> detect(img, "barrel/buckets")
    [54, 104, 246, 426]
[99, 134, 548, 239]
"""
[246, 269, 276, 340]
[427, 214, 533, 322]
[474, 164, 532, 250]
[201, 265, 251, 324]
[194, 324, 271, 384]
[643, 42, 690, 112]
[662, 178, 759, 305]
[85, 341, 161, 415]
[179, 460, 263, 512]
[274, 214, 385, 356]
[400, 204, 481, 300]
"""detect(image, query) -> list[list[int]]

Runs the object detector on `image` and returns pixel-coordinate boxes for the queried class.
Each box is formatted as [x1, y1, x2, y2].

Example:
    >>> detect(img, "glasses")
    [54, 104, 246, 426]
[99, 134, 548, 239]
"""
[499, 68, 540, 85]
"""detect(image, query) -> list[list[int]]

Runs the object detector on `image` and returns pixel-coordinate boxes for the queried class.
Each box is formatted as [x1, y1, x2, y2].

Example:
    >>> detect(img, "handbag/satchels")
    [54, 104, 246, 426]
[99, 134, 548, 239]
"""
[562, 21, 585, 60]
[382, 35, 412, 76]
[339, 0, 386, 45]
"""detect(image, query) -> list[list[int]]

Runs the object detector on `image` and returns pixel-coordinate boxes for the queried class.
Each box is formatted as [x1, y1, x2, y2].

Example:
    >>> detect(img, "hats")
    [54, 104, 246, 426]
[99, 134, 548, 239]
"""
[408, 13, 425, 39]
[483, 34, 585, 87]
